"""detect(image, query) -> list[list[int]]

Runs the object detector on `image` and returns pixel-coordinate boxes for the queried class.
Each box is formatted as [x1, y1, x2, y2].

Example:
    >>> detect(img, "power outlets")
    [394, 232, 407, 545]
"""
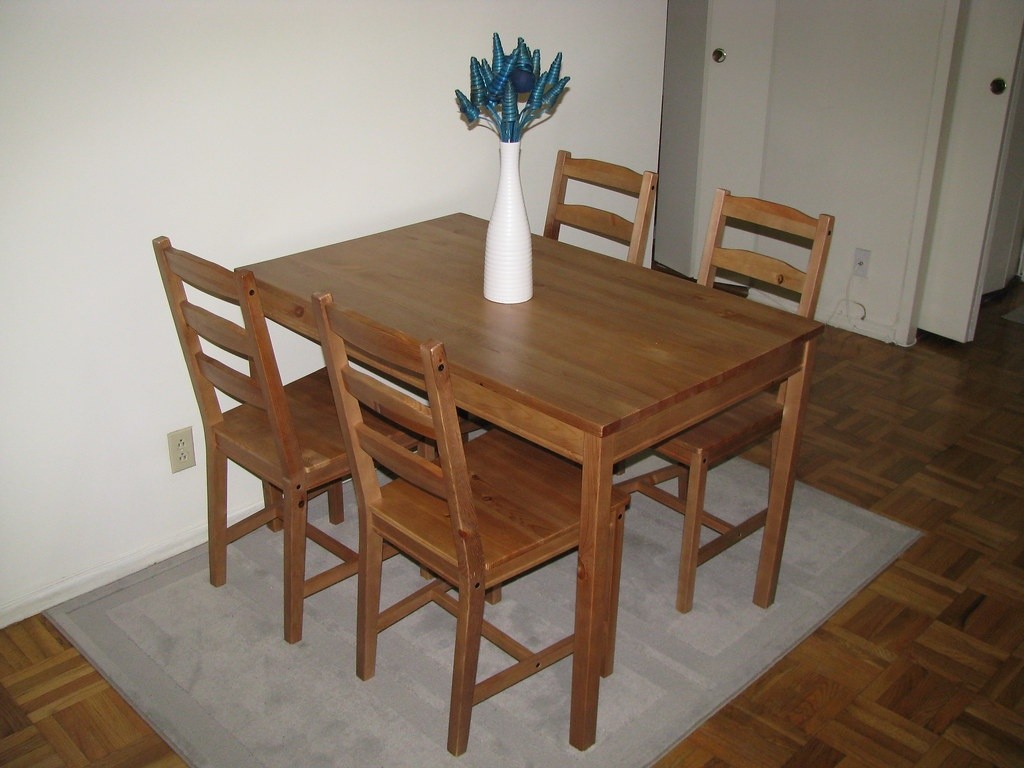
[852, 246, 871, 280]
[166, 427, 197, 473]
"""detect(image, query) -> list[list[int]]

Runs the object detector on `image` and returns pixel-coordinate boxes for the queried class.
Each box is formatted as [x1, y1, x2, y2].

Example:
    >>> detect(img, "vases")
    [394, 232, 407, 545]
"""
[483, 142, 534, 304]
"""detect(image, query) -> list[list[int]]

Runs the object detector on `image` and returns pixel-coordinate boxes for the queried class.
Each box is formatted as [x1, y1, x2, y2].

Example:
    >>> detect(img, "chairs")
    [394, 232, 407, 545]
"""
[154, 151, 834, 757]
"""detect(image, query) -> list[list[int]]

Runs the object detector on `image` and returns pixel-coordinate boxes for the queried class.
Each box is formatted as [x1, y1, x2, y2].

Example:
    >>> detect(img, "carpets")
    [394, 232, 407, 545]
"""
[42, 441, 922, 768]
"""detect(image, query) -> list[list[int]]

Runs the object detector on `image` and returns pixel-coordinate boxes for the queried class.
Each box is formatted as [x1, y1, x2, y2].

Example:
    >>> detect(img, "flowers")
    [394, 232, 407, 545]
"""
[457, 32, 571, 141]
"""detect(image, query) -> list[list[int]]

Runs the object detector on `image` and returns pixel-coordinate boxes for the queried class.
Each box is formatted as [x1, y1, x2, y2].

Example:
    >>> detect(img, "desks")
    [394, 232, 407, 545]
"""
[233, 213, 825, 750]
